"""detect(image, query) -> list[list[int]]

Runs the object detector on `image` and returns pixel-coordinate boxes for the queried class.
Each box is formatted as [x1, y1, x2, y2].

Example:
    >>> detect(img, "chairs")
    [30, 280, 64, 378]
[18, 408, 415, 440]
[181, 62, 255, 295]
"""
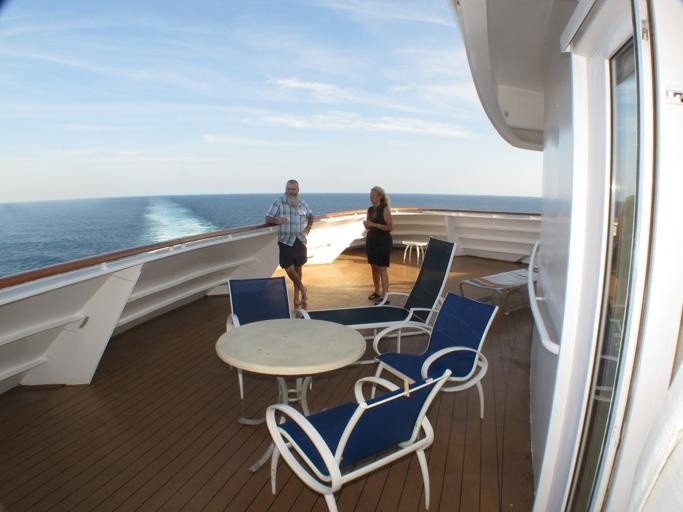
[302, 237, 456, 364]
[219, 277, 292, 399]
[266, 365, 465, 511]
[373, 290, 502, 423]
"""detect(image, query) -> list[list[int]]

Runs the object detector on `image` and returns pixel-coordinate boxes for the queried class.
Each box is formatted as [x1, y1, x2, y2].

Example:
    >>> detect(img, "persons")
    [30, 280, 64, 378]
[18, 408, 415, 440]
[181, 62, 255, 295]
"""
[362, 187, 393, 305]
[264, 180, 314, 310]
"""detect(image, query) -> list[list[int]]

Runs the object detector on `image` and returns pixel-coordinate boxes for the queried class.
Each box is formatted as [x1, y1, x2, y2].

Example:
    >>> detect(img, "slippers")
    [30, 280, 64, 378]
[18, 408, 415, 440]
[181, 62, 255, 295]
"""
[367, 293, 390, 305]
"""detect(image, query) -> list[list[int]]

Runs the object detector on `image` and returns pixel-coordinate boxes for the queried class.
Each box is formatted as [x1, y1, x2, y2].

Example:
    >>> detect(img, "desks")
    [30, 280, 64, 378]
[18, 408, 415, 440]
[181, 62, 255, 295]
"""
[213, 317, 368, 473]
[401, 239, 429, 265]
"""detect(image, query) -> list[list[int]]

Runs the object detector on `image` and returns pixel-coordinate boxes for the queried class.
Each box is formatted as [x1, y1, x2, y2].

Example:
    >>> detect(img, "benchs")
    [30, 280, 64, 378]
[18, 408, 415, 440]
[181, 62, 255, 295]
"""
[459, 263, 538, 316]
